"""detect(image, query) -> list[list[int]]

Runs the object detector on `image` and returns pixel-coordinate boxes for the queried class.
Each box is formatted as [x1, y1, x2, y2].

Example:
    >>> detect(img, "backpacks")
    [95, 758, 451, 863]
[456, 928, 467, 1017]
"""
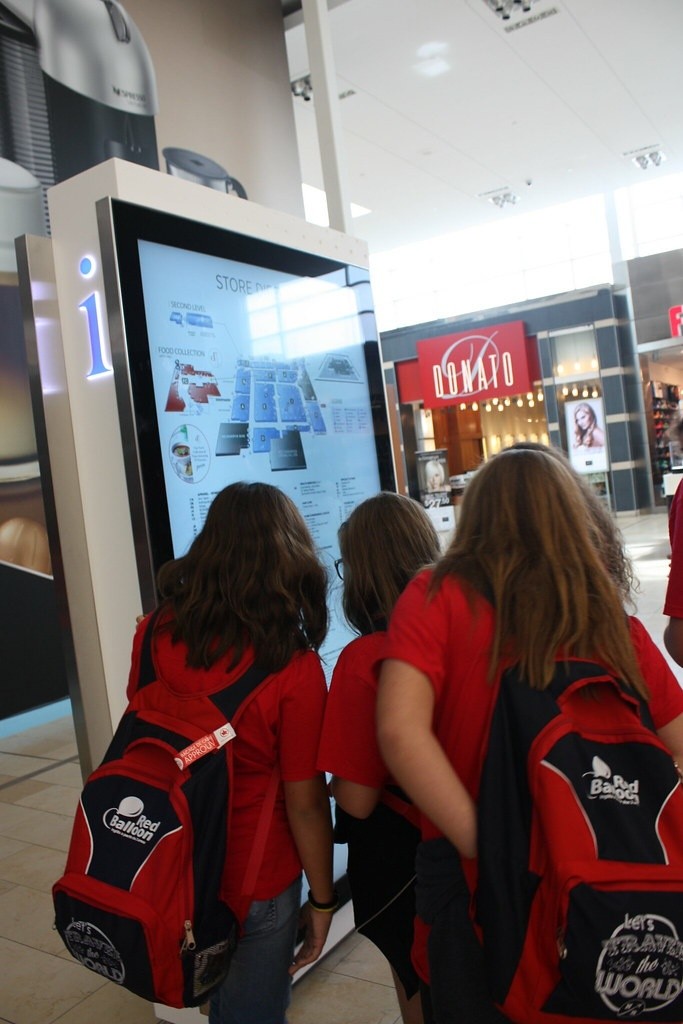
[48, 599, 300, 1013]
[464, 606, 683, 1024]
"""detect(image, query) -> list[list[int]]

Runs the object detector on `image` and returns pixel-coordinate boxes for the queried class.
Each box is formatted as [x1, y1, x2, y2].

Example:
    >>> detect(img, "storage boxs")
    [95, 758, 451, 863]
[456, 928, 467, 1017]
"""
[424, 505, 456, 532]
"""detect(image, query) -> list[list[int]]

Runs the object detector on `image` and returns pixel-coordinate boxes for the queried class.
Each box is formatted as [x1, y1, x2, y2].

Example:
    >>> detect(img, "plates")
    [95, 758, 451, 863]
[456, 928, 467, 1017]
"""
[170, 432, 193, 478]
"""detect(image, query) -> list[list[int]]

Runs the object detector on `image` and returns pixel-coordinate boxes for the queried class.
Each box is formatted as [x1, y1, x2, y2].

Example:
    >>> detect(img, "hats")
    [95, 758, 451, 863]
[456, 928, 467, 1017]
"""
[666, 399, 683, 440]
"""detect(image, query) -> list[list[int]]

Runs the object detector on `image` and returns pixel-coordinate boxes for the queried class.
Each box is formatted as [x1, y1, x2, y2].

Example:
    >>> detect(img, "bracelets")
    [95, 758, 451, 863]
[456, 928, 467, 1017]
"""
[307, 889, 341, 913]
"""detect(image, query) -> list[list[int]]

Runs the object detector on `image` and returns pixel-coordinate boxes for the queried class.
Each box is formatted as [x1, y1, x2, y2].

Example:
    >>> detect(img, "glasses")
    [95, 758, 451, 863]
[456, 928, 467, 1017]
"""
[333, 557, 346, 580]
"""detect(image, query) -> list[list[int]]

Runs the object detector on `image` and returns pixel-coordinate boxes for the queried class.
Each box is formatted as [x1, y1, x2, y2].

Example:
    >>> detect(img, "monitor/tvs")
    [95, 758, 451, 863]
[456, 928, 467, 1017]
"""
[96, 198, 398, 690]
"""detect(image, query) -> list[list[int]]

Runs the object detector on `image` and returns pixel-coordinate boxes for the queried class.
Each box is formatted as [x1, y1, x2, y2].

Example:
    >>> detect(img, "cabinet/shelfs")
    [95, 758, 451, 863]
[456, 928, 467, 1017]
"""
[652, 397, 680, 483]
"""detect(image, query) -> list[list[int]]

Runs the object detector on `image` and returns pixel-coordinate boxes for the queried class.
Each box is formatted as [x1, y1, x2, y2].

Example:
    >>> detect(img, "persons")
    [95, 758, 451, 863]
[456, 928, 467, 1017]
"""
[120, 482, 332, 1023]
[425, 461, 451, 494]
[573, 402, 606, 449]
[323, 490, 443, 1023]
[369, 397, 683, 1023]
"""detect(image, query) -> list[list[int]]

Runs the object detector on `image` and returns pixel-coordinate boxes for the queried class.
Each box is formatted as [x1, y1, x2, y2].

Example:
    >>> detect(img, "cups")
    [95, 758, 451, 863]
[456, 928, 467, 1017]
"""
[170, 440, 190, 465]
